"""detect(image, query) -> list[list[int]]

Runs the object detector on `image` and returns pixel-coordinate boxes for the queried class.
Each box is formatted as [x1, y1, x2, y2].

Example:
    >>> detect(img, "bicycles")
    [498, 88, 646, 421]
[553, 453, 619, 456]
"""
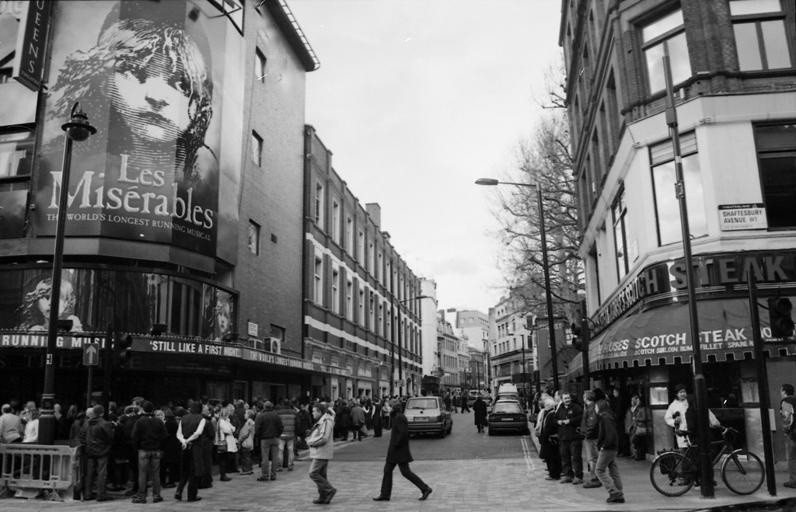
[650, 423, 766, 497]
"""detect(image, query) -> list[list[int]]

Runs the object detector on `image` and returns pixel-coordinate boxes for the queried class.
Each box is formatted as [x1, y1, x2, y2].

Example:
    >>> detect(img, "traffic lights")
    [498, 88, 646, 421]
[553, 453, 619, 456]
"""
[768, 297, 795, 338]
[571, 320, 587, 351]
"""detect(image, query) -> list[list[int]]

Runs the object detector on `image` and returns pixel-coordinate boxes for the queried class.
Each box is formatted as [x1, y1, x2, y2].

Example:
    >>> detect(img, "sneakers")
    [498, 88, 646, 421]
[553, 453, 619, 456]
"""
[72, 493, 114, 501]
[678, 474, 718, 492]
[132, 497, 146, 503]
[174, 494, 183, 501]
[216, 464, 254, 483]
[271, 476, 276, 480]
[187, 496, 202, 502]
[152, 496, 164, 502]
[110, 479, 178, 492]
[341, 432, 382, 441]
[783, 482, 795, 488]
[544, 460, 603, 488]
[322, 488, 336, 503]
[605, 492, 624, 503]
[312, 498, 332, 504]
[256, 476, 270, 481]
[418, 487, 432, 500]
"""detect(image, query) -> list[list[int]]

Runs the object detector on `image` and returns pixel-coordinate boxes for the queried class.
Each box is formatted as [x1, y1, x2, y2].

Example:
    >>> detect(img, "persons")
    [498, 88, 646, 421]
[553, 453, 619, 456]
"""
[1, 396, 283, 502]
[35, 17, 219, 189]
[443, 392, 470, 414]
[276, 392, 415, 470]
[204, 295, 233, 342]
[780, 384, 796, 489]
[305, 404, 337, 505]
[473, 396, 489, 433]
[372, 404, 432, 503]
[520, 386, 723, 503]
[415, 393, 419, 397]
[14, 277, 83, 333]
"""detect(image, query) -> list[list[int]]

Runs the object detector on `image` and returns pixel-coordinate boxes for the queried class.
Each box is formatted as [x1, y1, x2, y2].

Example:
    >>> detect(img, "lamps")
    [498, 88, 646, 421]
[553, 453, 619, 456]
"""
[150, 324, 169, 336]
[57, 319, 73, 332]
[222, 332, 240, 342]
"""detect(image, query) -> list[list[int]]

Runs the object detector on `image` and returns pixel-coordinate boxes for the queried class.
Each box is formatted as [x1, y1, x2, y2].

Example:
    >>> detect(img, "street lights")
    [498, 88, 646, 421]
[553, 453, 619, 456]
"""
[508, 333, 526, 409]
[475, 178, 559, 394]
[463, 352, 491, 394]
[398, 295, 427, 410]
[27, 101, 98, 498]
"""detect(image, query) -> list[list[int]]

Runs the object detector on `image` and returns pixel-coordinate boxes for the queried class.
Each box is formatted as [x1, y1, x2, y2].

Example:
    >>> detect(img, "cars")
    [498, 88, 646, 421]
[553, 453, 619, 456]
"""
[402, 396, 453, 438]
[446, 381, 531, 408]
[487, 399, 528, 436]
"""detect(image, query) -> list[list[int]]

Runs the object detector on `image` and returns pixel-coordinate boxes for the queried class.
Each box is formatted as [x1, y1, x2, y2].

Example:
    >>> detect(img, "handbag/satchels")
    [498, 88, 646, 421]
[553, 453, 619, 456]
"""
[213, 443, 228, 457]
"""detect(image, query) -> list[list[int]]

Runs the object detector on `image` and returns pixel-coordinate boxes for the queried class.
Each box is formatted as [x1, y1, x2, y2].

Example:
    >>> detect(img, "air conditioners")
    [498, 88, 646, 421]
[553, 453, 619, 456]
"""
[248, 339, 263, 349]
[262, 336, 282, 354]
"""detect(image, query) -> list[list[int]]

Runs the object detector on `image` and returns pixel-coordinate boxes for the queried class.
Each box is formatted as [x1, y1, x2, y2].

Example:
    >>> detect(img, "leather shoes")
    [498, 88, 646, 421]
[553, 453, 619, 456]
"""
[372, 494, 392, 502]
